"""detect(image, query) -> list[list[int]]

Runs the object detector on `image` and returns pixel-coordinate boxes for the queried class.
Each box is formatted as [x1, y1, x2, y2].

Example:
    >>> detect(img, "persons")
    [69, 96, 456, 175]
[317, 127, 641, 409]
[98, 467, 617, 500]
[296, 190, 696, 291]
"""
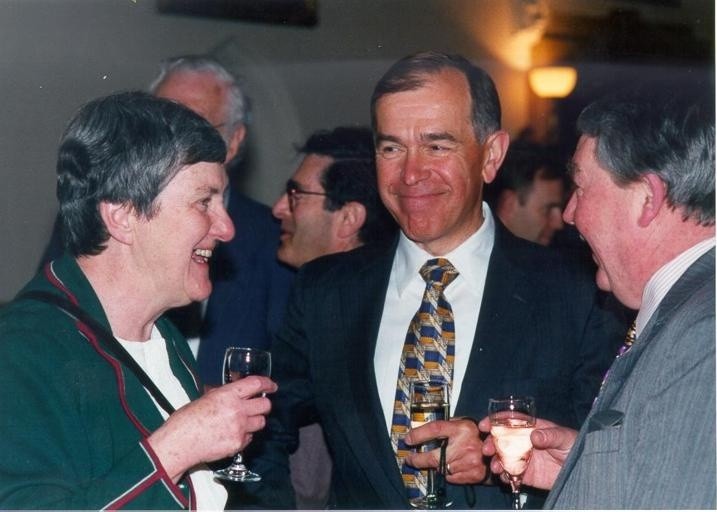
[271, 127, 393, 267]
[493, 140, 566, 248]
[246, 51, 630, 511]
[2, 91, 279, 512]
[44, 56, 296, 392]
[478, 62, 717, 512]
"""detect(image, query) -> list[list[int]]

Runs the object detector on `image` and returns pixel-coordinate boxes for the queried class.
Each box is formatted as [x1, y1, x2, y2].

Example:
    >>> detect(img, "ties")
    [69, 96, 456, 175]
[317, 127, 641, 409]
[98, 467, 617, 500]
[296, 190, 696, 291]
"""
[384, 254, 463, 508]
[591, 318, 640, 412]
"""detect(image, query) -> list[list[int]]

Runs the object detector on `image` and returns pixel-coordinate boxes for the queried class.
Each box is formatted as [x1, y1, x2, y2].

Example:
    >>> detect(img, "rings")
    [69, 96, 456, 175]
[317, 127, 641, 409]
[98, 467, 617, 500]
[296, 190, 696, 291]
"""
[447, 463, 453, 476]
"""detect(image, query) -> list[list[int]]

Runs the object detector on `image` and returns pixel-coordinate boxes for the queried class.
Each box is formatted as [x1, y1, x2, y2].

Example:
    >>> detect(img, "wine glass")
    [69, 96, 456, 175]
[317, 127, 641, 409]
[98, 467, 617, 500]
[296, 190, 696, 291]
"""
[406, 368, 456, 507]
[488, 392, 538, 509]
[209, 347, 273, 482]
